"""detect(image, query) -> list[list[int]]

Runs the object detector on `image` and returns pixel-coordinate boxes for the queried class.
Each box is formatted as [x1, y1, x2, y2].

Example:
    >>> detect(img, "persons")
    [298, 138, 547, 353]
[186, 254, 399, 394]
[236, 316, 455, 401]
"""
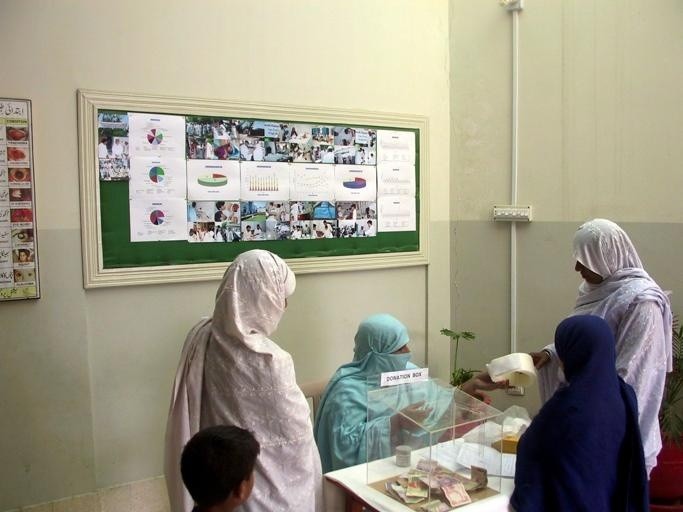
[315, 313, 509, 475]
[11, 249, 38, 284]
[186, 199, 378, 241]
[186, 117, 378, 164]
[180, 426, 260, 512]
[502, 314, 649, 511]
[528, 218, 674, 480]
[166, 248, 321, 512]
[95, 113, 131, 180]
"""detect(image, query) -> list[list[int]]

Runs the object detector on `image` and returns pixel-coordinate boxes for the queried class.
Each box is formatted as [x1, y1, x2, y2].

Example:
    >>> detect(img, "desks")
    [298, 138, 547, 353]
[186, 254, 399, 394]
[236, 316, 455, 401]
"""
[322, 436, 518, 512]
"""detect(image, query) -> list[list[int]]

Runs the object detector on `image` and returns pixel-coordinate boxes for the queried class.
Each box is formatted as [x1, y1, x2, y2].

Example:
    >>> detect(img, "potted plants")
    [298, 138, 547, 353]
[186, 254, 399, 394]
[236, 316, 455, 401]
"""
[647, 308, 683, 511]
[438, 326, 489, 440]
